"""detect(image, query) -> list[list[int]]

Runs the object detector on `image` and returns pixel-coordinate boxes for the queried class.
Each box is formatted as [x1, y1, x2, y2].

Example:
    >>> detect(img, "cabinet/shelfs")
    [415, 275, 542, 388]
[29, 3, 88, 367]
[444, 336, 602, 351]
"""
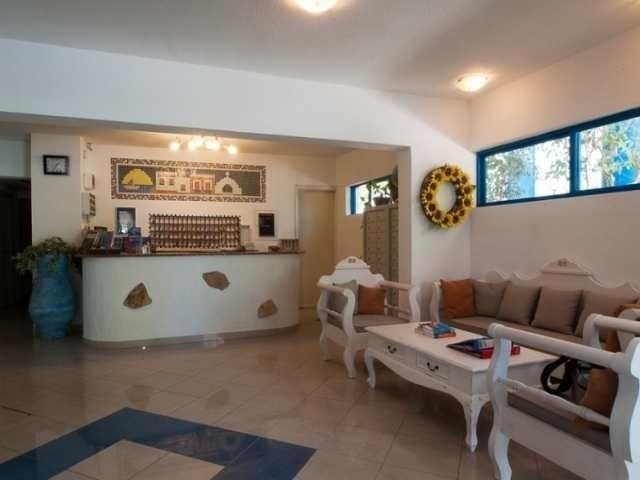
[389, 199, 401, 319]
[361, 202, 389, 301]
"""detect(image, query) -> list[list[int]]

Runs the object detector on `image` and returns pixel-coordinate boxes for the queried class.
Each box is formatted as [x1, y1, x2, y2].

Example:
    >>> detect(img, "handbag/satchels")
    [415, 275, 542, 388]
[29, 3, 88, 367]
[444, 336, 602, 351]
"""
[539, 353, 621, 431]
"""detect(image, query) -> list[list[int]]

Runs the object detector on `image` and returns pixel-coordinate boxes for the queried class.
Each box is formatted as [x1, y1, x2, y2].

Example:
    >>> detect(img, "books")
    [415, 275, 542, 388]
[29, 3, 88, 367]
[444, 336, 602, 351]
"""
[415, 321, 456, 339]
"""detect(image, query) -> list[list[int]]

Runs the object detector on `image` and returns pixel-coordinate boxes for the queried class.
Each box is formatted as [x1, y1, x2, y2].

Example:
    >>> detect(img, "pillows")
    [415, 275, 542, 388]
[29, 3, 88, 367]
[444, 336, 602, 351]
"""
[356, 283, 386, 316]
[439, 277, 640, 352]
[575, 326, 621, 436]
[329, 278, 358, 317]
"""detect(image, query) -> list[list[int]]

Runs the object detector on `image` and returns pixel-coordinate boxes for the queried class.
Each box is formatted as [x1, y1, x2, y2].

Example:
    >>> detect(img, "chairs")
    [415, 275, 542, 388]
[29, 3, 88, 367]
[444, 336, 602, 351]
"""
[313, 255, 421, 383]
[479, 312, 640, 479]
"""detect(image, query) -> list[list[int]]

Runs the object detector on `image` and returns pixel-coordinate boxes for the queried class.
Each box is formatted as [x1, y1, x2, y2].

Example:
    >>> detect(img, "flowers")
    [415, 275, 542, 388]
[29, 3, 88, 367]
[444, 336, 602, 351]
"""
[418, 162, 478, 233]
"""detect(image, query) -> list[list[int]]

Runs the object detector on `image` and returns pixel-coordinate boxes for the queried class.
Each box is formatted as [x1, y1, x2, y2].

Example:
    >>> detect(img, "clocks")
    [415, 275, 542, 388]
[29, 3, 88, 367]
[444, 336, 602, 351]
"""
[42, 153, 70, 177]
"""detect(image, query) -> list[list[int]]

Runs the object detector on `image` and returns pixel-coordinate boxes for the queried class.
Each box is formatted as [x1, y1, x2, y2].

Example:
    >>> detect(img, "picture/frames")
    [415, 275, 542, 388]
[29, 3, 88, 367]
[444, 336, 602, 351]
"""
[109, 156, 268, 204]
[115, 207, 136, 237]
[254, 209, 279, 242]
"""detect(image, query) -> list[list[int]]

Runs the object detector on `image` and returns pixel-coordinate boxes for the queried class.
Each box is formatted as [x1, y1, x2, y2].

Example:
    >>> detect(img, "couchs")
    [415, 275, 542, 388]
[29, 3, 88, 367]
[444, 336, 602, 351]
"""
[428, 254, 640, 366]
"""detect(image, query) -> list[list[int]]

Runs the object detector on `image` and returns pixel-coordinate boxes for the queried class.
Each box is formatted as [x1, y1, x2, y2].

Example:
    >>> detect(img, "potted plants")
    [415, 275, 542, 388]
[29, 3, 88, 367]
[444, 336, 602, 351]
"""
[11, 234, 83, 343]
[365, 175, 398, 208]
